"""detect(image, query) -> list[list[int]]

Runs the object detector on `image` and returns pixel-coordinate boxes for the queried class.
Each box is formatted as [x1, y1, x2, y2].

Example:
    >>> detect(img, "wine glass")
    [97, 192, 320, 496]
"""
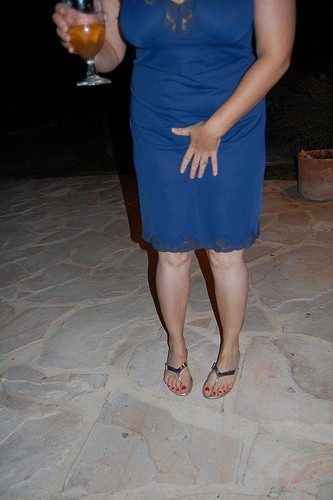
[62, 0, 111, 87]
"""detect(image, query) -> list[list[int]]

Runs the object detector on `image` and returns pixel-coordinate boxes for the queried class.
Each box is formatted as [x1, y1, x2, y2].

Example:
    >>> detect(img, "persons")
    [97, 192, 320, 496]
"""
[51, 0, 295, 401]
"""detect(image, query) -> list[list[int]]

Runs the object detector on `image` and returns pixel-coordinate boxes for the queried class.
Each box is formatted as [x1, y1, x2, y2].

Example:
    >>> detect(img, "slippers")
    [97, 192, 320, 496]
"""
[202, 363, 239, 399]
[165, 361, 194, 396]
